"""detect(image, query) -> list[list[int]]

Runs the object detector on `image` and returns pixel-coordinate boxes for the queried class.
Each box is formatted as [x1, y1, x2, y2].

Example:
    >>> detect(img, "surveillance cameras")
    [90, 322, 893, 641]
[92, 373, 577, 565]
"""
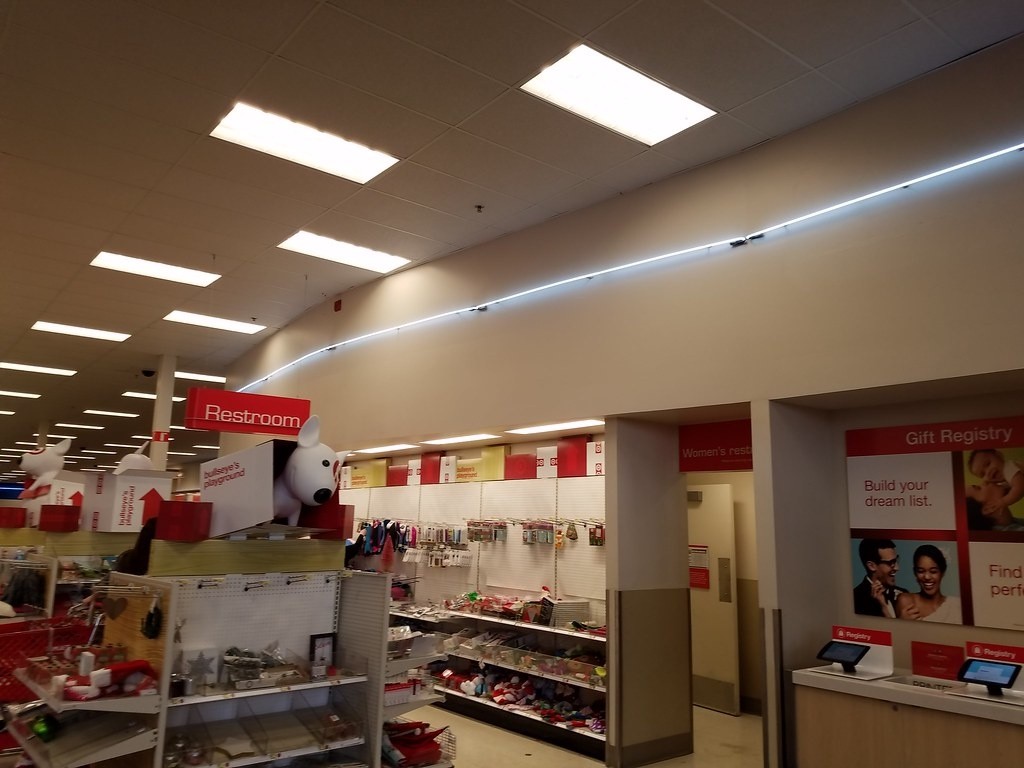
[142, 369, 156, 377]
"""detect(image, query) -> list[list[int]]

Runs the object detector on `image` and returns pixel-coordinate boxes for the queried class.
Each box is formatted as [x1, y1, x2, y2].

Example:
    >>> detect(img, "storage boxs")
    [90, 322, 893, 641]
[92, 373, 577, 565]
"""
[24, 468, 84, 527]
[74, 467, 182, 532]
[384, 683, 412, 707]
[199, 438, 360, 539]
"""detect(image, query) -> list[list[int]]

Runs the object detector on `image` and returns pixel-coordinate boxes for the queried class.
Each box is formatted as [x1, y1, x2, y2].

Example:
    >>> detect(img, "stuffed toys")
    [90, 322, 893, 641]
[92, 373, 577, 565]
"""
[256, 415, 352, 530]
[517, 639, 606, 686]
[16, 438, 72, 492]
[428, 658, 605, 737]
[113, 441, 154, 479]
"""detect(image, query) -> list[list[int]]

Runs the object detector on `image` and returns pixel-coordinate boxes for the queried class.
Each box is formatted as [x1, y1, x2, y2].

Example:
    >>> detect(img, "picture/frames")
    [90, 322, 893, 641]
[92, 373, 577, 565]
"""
[310, 633, 338, 666]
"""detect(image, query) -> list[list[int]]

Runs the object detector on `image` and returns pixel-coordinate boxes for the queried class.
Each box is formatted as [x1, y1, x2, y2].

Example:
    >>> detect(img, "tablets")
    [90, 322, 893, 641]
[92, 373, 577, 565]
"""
[816, 640, 871, 665]
[957, 659, 1022, 689]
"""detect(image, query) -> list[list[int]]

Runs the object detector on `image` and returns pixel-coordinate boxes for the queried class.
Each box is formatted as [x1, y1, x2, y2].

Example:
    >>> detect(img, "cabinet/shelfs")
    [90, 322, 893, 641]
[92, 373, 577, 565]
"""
[0, 531, 607, 768]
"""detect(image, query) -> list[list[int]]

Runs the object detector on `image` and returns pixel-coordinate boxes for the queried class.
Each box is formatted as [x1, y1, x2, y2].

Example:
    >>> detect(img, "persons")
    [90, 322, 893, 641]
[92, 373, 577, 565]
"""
[963, 449, 1024, 532]
[851, 537, 963, 625]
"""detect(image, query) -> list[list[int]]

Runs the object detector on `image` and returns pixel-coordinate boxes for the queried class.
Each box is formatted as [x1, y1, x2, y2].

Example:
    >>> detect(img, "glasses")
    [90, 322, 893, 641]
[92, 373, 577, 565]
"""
[880, 554, 900, 568]
